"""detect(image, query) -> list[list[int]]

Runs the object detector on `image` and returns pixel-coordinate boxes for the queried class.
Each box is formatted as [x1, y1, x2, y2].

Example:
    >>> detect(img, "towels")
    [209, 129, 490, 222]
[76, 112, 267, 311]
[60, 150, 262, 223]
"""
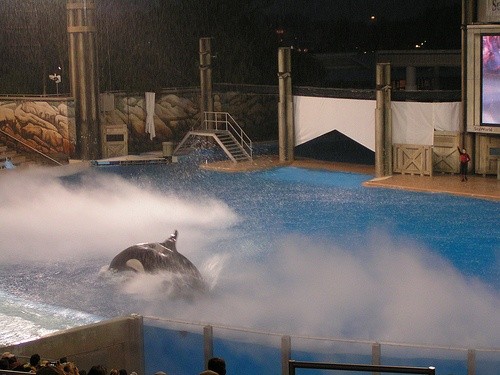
[144, 93, 156, 141]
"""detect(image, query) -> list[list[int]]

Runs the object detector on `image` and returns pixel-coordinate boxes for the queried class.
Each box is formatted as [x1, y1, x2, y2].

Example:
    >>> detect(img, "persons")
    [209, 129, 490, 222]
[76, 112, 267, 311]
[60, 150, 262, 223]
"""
[457, 145, 470, 182]
